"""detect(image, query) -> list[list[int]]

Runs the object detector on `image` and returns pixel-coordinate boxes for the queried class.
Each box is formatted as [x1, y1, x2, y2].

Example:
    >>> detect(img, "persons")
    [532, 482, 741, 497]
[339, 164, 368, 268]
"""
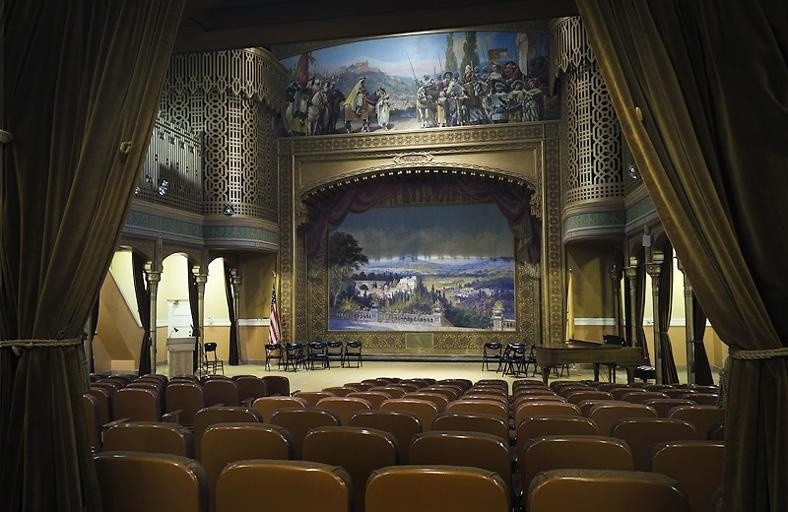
[416, 61, 545, 128]
[286, 76, 391, 132]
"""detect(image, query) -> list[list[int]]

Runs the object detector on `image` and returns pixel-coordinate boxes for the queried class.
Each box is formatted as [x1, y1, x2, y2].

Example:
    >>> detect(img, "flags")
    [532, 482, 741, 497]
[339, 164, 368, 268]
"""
[268, 283, 281, 345]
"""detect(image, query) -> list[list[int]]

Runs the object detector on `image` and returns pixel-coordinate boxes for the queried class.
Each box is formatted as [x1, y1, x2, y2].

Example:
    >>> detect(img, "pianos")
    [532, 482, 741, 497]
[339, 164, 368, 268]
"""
[535, 338, 642, 385]
